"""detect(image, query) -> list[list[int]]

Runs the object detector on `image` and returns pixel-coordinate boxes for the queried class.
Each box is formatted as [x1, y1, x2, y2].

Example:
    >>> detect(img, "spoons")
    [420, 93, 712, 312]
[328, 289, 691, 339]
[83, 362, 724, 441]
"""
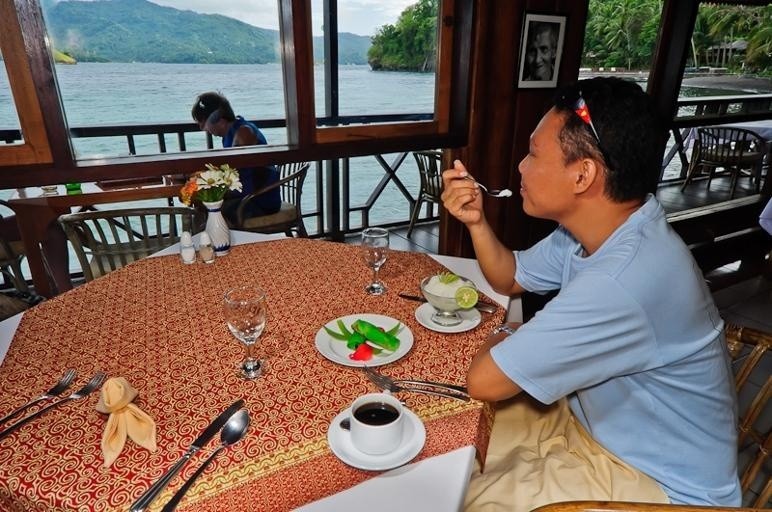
[464, 175, 512, 198]
[160, 409, 251, 511]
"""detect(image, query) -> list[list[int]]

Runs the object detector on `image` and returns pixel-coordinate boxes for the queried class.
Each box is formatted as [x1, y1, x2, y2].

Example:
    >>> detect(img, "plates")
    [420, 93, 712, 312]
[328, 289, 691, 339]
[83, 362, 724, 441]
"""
[416, 302, 482, 334]
[314, 313, 414, 368]
[328, 405, 427, 471]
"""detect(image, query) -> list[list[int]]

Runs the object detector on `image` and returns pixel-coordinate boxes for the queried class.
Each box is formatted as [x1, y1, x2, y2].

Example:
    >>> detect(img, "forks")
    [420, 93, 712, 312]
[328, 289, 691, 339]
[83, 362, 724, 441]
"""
[358, 368, 472, 404]
[0, 367, 107, 437]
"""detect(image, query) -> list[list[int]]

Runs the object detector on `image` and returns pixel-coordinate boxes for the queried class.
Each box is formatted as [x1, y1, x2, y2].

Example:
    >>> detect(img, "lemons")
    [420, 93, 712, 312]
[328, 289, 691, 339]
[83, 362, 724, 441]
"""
[456, 287, 479, 309]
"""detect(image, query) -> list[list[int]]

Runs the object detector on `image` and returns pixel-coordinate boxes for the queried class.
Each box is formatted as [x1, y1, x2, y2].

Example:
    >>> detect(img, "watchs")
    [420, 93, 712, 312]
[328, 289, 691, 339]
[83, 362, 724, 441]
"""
[491, 324, 517, 336]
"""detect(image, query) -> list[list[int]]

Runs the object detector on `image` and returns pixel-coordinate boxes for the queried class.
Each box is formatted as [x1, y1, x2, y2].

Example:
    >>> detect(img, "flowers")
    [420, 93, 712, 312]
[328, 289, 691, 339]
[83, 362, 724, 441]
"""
[179, 163, 244, 205]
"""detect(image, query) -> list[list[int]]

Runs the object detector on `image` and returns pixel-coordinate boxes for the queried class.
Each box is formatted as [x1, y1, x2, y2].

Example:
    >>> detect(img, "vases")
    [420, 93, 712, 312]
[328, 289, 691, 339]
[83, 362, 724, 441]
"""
[202, 199, 231, 255]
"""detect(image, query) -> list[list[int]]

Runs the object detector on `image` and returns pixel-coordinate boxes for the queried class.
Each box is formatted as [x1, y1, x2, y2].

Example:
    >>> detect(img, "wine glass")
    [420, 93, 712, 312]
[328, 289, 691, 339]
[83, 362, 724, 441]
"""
[421, 275, 469, 326]
[361, 228, 389, 295]
[223, 287, 272, 380]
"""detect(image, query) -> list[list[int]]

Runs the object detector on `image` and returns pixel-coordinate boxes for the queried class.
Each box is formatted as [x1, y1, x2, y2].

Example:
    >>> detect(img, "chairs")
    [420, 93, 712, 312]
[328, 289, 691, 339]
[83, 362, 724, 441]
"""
[680, 126, 764, 199]
[57, 206, 202, 283]
[531, 323, 772, 512]
[0, 199, 30, 293]
[236, 161, 311, 237]
[406, 149, 442, 239]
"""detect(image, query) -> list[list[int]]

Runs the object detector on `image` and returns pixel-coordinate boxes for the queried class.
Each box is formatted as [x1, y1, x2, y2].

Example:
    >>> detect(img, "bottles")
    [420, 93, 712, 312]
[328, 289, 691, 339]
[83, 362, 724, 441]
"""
[179, 231, 214, 264]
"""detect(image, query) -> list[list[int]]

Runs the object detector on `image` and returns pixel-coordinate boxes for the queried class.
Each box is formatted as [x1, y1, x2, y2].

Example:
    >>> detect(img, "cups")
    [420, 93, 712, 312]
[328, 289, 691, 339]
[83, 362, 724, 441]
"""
[350, 388, 404, 456]
[162, 174, 176, 187]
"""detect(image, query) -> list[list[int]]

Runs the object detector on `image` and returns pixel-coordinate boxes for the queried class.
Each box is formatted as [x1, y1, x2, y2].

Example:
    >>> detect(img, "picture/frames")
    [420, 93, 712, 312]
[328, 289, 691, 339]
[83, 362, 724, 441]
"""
[513, 8, 570, 91]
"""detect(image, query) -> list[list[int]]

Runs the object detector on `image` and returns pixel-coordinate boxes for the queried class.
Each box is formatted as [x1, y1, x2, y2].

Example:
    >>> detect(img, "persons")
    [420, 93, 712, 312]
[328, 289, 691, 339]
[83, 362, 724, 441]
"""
[191, 92, 281, 232]
[441, 76, 742, 512]
[523, 26, 557, 80]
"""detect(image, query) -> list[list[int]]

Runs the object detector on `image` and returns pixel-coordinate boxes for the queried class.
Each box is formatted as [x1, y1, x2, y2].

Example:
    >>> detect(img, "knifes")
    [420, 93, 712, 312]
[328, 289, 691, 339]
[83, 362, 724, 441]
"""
[398, 294, 498, 314]
[129, 401, 245, 511]
